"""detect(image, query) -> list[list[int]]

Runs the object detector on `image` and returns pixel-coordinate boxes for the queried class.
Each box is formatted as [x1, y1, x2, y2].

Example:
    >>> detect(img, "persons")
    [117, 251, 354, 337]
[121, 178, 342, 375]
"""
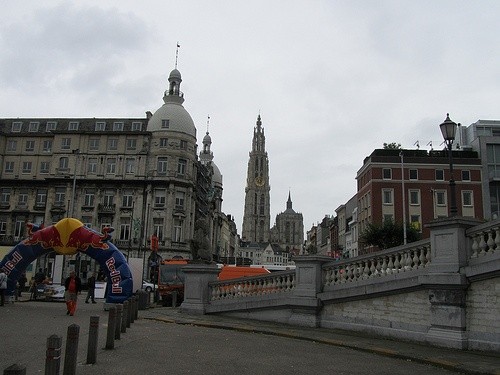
[64, 270, 82, 316]
[84, 273, 98, 304]
[8, 268, 53, 303]
[0, 269, 8, 306]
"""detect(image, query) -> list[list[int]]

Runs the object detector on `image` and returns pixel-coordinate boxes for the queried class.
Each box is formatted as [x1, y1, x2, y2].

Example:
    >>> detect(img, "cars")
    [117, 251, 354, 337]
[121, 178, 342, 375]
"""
[143, 280, 158, 293]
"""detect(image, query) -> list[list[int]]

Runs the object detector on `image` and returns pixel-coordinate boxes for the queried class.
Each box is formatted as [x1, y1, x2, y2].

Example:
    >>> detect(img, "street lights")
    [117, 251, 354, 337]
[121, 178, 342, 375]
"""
[439, 113, 459, 216]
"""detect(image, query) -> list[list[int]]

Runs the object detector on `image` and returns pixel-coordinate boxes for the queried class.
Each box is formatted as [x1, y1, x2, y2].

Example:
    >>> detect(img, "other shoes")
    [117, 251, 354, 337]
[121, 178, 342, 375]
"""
[85, 301, 89, 303]
[92, 301, 97, 303]
[67, 310, 70, 315]
